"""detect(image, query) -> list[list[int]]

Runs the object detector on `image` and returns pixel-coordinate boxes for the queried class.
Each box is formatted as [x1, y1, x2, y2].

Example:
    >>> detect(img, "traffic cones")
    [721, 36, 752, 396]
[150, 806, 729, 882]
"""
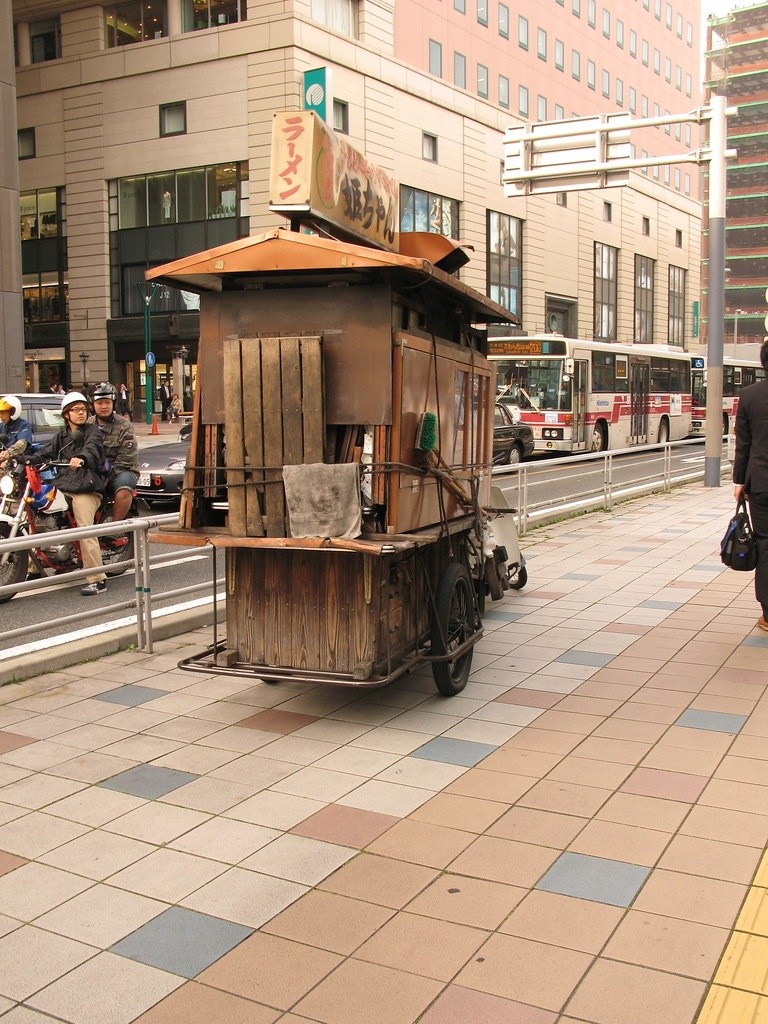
[147, 414, 159, 435]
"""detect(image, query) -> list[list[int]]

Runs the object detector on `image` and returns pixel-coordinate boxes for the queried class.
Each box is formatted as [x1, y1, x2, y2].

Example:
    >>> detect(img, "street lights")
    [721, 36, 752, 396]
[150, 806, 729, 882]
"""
[78, 351, 90, 389]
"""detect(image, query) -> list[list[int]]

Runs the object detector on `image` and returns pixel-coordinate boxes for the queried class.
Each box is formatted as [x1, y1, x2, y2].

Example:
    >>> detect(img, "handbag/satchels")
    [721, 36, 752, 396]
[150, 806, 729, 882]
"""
[720, 493, 757, 572]
[51, 466, 105, 494]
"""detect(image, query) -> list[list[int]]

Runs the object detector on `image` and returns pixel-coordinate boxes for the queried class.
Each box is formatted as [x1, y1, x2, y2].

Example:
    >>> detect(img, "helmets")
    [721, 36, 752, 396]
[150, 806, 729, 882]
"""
[88, 382, 118, 416]
[61, 392, 87, 412]
[0, 396, 22, 421]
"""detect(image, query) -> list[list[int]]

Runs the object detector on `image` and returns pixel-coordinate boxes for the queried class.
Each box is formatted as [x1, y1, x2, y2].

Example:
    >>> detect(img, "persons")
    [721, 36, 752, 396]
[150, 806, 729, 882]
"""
[118, 383, 133, 421]
[87, 381, 140, 542]
[17, 391, 109, 594]
[81, 382, 92, 397]
[160, 380, 173, 422]
[732, 340, 768, 632]
[169, 394, 182, 423]
[48, 383, 74, 395]
[0, 396, 35, 522]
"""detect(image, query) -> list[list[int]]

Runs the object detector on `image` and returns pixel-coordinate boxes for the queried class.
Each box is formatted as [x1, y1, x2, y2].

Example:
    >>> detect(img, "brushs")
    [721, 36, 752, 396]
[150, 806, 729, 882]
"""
[415, 412, 487, 515]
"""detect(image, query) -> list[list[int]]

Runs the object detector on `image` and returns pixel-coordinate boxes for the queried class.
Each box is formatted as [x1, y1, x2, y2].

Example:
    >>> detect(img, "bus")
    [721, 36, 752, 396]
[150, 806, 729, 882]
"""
[690, 353, 766, 444]
[487, 331, 694, 462]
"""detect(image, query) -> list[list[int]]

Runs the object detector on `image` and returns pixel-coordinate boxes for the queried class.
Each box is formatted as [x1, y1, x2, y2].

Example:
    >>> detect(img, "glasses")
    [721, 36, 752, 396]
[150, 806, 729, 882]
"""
[67, 406, 87, 412]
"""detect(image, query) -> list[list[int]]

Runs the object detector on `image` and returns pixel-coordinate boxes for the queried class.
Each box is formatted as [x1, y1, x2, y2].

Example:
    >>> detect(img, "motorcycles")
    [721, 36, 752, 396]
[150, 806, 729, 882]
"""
[0, 433, 138, 605]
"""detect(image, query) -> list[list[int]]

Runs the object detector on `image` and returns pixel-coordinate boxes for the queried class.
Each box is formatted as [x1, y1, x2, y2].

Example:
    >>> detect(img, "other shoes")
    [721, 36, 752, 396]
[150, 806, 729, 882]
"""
[102, 532, 125, 540]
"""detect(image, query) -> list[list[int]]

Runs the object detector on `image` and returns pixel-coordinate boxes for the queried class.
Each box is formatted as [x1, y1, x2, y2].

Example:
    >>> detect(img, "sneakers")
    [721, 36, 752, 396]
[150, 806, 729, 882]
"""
[80, 579, 108, 595]
[25, 572, 42, 581]
[758, 616, 768, 630]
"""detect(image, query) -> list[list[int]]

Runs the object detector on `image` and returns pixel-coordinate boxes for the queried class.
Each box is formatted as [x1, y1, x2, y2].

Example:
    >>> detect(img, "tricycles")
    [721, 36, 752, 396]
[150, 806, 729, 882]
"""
[140, 224, 529, 698]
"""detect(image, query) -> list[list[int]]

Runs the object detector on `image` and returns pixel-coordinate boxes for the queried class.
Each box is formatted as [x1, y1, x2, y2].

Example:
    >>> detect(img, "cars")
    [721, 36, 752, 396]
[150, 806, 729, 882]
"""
[491, 402, 535, 475]
[133, 421, 194, 510]
[0, 392, 67, 448]
